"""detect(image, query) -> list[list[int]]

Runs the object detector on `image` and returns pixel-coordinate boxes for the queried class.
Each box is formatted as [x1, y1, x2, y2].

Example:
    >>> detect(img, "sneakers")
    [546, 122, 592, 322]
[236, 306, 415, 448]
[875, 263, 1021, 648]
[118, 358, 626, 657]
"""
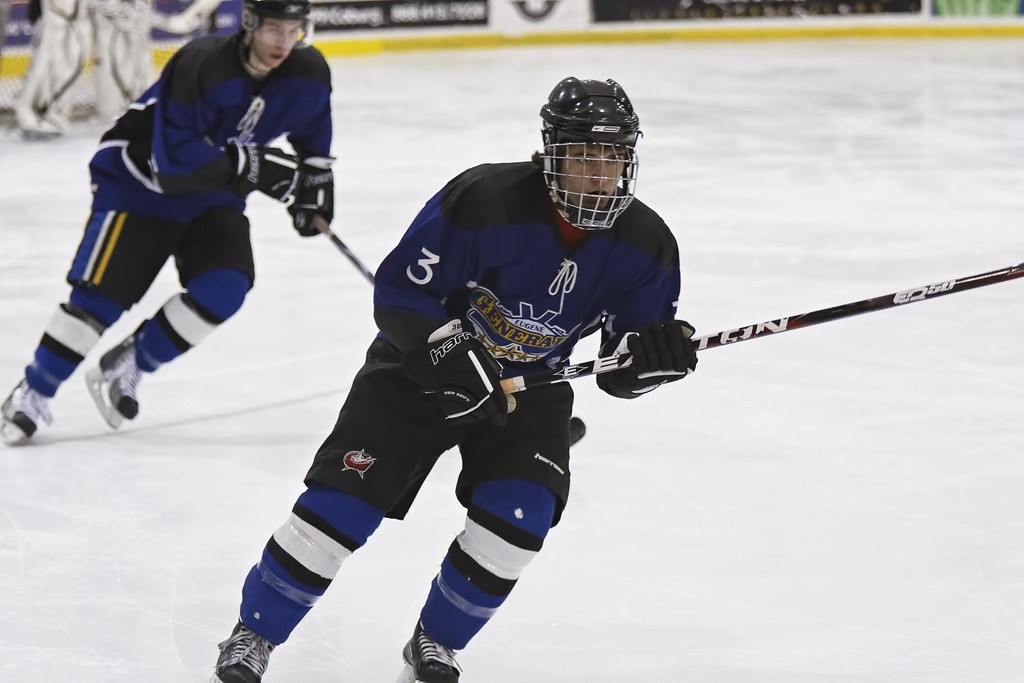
[99, 333, 140, 420]
[1, 378, 52, 438]
[403, 619, 462, 683]
[215, 617, 276, 683]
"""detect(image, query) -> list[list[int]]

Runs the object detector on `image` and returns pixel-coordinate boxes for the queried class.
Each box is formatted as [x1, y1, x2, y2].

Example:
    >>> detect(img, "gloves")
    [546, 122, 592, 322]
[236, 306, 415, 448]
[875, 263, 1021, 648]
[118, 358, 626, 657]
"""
[226, 140, 301, 203]
[596, 321, 698, 399]
[287, 154, 336, 237]
[411, 319, 508, 425]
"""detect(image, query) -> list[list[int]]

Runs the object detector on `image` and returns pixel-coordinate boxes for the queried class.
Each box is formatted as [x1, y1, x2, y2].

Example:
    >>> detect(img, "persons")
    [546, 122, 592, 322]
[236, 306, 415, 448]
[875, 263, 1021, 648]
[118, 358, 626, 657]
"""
[211, 78, 699, 683]
[7, 0, 162, 140]
[0, 1, 335, 449]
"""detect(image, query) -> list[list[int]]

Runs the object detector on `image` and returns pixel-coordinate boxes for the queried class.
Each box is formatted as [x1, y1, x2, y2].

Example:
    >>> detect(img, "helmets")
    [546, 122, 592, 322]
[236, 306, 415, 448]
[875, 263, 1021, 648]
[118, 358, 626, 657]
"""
[539, 76, 639, 231]
[242, 0, 316, 50]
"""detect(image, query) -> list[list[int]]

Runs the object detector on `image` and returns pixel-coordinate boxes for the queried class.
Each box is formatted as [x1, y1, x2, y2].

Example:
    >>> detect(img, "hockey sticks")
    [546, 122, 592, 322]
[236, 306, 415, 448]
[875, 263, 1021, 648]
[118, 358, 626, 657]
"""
[311, 210, 374, 286]
[501, 263, 1023, 398]
